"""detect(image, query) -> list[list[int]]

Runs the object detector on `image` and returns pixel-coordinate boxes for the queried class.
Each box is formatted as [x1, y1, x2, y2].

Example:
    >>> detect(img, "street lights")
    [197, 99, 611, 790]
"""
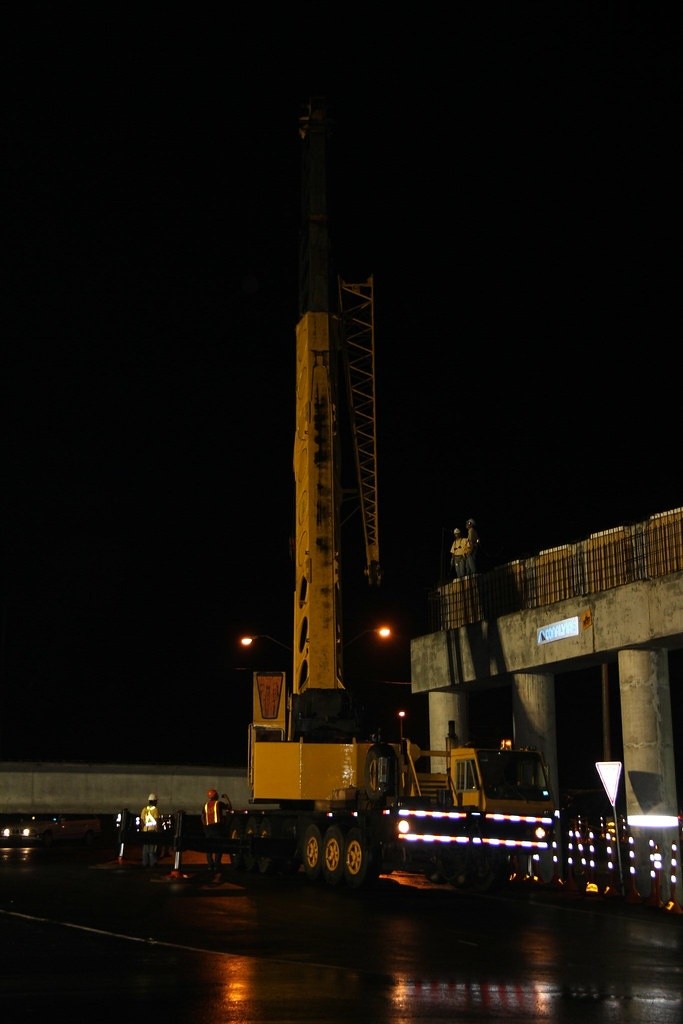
[398, 710, 406, 746]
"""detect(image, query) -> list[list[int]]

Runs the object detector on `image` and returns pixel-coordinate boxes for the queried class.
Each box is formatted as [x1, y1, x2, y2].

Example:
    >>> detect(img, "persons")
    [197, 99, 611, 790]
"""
[203, 788, 233, 873]
[139, 792, 162, 867]
[466, 518, 480, 574]
[450, 528, 468, 576]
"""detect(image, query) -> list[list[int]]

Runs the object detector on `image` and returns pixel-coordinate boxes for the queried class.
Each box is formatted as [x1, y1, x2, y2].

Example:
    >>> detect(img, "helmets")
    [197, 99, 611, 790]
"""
[148, 793, 156, 800]
[207, 789, 217, 799]
[453, 528, 460, 534]
[467, 518, 476, 526]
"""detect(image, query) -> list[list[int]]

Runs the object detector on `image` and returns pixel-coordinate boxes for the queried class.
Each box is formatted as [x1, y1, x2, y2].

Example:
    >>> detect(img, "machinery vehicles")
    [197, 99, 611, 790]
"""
[117, 88, 560, 896]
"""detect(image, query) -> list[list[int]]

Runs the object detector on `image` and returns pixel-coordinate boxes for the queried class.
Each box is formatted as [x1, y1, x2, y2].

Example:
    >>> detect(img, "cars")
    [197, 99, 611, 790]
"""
[0, 813, 102, 848]
